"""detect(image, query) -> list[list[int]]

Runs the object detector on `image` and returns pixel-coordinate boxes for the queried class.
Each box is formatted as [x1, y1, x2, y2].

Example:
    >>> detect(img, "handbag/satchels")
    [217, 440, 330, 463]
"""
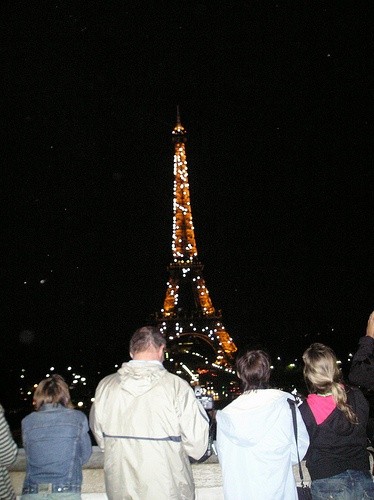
[297, 485, 312, 500]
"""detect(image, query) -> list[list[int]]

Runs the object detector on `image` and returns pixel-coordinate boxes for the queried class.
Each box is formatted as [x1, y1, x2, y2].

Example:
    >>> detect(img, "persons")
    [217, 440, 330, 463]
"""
[215, 351, 310, 500]
[88, 326, 209, 500]
[299, 311, 374, 500]
[20, 374, 93, 500]
[0, 404, 18, 500]
